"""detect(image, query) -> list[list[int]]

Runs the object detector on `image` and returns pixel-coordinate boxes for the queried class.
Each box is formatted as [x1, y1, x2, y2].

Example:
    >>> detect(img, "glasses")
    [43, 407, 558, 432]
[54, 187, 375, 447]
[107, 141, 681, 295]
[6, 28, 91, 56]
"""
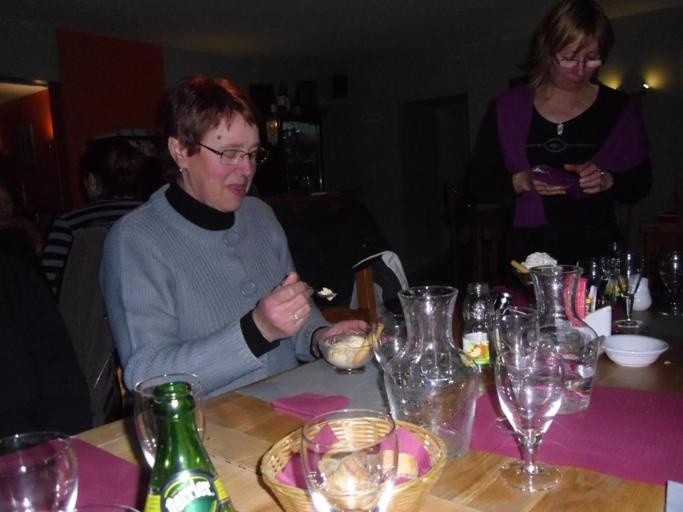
[195, 142, 271, 168]
[553, 51, 603, 70]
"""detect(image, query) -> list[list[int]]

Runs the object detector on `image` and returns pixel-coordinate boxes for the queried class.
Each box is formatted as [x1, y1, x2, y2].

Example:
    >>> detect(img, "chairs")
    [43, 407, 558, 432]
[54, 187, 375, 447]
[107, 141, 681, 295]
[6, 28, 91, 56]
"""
[37, 199, 149, 434]
[306, 265, 377, 327]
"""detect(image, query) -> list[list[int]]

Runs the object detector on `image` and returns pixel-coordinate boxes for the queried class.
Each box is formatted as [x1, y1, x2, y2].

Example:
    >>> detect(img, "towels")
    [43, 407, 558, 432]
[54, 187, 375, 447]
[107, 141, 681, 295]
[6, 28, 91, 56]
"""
[272, 392, 349, 420]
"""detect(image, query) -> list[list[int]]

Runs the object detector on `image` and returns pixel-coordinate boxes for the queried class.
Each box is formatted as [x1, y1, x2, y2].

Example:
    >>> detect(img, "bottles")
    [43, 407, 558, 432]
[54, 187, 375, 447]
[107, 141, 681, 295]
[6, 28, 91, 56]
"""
[526, 263, 602, 417]
[379, 285, 479, 457]
[143, 382, 235, 512]
[465, 281, 495, 332]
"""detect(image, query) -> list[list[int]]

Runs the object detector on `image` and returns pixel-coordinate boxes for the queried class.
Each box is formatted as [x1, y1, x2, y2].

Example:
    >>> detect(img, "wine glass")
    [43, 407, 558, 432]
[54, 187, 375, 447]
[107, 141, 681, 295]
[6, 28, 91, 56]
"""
[490, 344, 568, 495]
[580, 249, 683, 328]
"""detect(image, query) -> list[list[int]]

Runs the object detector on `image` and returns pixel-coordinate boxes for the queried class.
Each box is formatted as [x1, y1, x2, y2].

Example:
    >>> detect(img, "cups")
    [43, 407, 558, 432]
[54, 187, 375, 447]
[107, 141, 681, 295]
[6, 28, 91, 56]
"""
[131, 374, 206, 468]
[492, 304, 540, 367]
[0, 430, 81, 512]
[373, 314, 421, 383]
[300, 406, 399, 511]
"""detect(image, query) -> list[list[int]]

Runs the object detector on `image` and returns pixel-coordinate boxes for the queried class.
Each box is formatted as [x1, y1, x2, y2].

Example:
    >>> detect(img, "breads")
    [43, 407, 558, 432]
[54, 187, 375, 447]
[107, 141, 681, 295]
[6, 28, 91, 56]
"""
[382, 449, 420, 479]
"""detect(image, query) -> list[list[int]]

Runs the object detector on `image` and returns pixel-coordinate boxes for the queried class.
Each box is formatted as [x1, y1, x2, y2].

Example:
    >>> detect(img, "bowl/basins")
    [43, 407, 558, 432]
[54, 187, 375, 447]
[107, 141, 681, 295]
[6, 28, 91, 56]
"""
[599, 333, 670, 368]
[319, 334, 371, 374]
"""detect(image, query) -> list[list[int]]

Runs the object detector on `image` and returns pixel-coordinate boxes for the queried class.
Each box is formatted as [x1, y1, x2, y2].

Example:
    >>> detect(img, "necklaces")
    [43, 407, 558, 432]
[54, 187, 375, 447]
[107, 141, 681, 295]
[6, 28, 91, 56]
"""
[542, 87, 579, 136]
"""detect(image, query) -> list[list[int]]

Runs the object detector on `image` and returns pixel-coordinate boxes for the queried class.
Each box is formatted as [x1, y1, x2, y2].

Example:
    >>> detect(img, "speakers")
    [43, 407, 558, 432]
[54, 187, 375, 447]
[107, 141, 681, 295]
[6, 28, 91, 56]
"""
[331, 72, 348, 98]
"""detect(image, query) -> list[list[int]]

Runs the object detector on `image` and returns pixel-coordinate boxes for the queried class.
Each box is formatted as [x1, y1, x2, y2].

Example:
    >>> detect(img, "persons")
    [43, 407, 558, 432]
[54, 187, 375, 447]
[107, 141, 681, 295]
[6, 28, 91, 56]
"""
[0, 186, 42, 256]
[41, 139, 145, 299]
[98, 69, 369, 401]
[465, 0, 654, 265]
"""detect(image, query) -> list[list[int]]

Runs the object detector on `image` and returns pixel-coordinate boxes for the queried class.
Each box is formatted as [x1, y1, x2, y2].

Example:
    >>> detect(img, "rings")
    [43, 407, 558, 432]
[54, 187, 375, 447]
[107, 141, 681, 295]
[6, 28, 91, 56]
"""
[293, 314, 299, 320]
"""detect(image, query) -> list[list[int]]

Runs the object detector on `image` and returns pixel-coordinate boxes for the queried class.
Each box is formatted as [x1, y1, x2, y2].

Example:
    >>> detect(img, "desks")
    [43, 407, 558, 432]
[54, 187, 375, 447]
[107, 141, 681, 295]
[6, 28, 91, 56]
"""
[638, 209, 682, 300]
